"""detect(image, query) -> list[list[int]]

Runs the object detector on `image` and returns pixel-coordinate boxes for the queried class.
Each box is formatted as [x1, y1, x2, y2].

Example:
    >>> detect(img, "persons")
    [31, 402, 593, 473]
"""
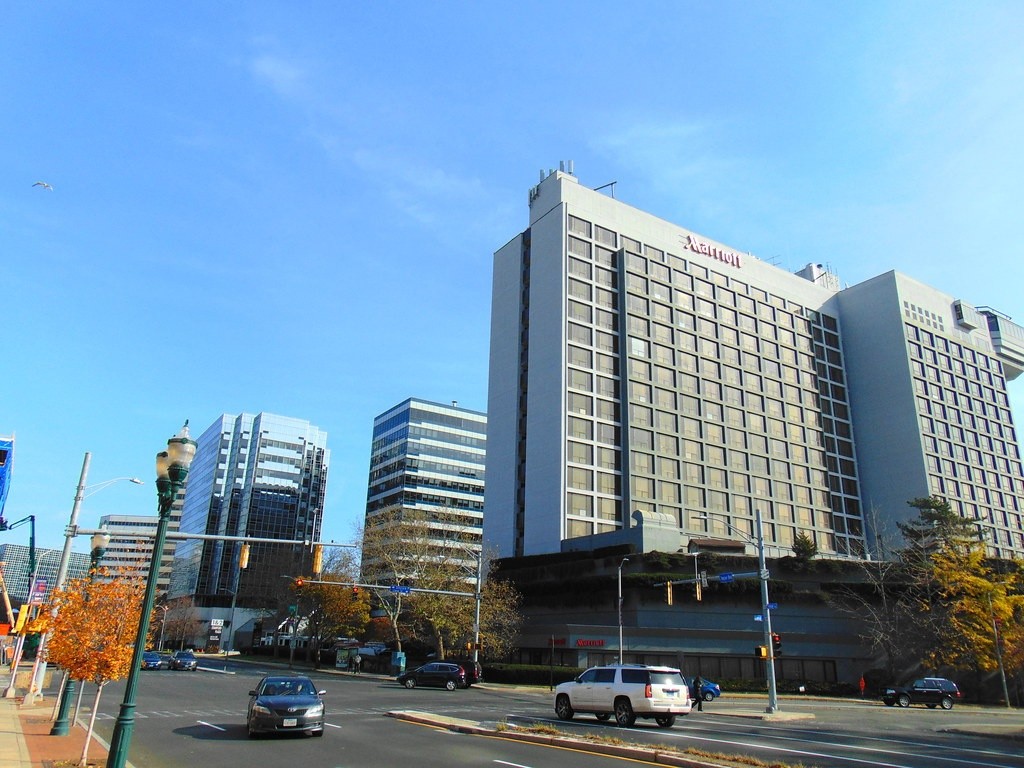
[692, 675, 704, 711]
[354, 654, 361, 674]
[348, 657, 355, 673]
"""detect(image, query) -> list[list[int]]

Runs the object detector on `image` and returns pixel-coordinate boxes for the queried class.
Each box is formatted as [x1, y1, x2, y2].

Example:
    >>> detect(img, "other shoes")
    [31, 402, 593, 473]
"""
[698, 710, 702, 711]
[691, 707, 693, 711]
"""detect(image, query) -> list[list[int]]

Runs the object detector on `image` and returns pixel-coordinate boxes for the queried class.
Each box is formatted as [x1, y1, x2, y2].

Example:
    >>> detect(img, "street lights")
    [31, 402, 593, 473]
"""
[103, 419, 198, 768]
[49, 522, 111, 737]
[220, 587, 236, 671]
[453, 542, 483, 662]
[988, 580, 1012, 708]
[33, 476, 142, 693]
[619, 558, 630, 664]
[691, 515, 779, 711]
[155, 604, 166, 650]
[8, 544, 79, 672]
[282, 575, 299, 663]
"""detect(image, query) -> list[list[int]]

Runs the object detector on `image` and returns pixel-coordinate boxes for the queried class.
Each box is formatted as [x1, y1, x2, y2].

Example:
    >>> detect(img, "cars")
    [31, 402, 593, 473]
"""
[246, 676, 327, 737]
[140, 652, 162, 670]
[684, 677, 720, 701]
[168, 651, 198, 671]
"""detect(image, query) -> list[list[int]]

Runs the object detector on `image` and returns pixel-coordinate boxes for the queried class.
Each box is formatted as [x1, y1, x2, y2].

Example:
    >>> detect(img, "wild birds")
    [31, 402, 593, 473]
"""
[33, 182, 53, 192]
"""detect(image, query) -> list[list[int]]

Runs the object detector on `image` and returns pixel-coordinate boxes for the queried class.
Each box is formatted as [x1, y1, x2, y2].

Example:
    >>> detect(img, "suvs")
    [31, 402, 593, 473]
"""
[396, 661, 467, 692]
[553, 663, 692, 728]
[406, 657, 483, 689]
[880, 676, 961, 710]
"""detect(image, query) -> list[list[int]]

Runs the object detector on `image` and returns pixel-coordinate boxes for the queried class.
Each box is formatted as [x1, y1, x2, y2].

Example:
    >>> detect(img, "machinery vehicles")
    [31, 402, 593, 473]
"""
[0, 561, 43, 657]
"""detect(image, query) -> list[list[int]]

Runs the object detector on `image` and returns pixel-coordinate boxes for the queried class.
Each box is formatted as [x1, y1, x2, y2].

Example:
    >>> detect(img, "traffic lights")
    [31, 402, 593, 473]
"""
[692, 581, 701, 601]
[664, 582, 673, 606]
[297, 580, 302, 596]
[473, 643, 483, 649]
[353, 588, 358, 602]
[754, 645, 766, 656]
[771, 633, 783, 658]
